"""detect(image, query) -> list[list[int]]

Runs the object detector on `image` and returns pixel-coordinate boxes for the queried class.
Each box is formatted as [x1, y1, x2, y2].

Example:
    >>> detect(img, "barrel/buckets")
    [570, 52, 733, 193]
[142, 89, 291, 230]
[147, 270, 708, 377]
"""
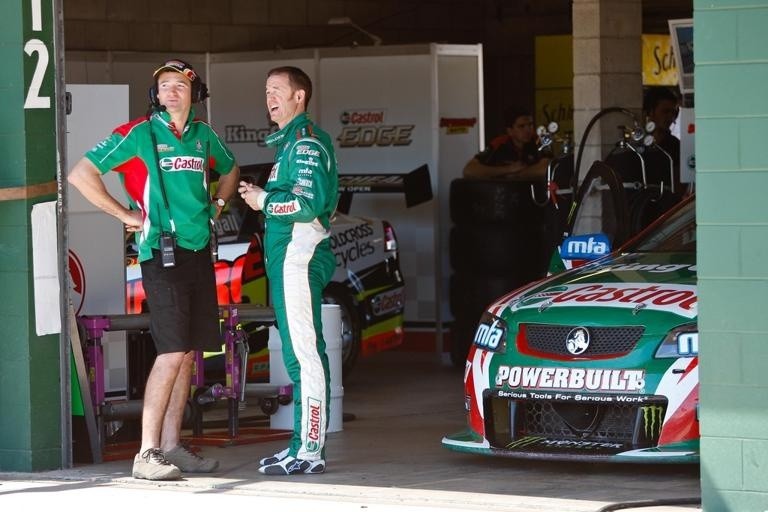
[268, 303, 345, 433]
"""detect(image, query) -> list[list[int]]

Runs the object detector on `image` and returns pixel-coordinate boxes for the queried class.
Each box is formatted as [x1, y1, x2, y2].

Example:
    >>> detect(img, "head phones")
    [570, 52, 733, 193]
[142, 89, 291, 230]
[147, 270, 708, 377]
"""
[149, 65, 207, 105]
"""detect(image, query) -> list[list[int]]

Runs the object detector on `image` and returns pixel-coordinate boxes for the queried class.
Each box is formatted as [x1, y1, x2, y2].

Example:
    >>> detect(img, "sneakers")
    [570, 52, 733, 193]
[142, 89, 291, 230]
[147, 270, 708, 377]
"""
[166, 446, 217, 472]
[258, 448, 325, 474]
[132, 449, 181, 480]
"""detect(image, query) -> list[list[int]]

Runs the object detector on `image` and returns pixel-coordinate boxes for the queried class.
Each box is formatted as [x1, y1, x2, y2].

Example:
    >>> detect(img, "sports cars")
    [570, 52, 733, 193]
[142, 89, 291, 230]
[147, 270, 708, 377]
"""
[440, 157, 700, 471]
[122, 159, 436, 403]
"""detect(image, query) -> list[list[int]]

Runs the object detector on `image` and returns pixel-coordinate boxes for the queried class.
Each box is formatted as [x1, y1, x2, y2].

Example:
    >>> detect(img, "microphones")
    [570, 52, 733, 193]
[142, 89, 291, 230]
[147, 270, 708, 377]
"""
[155, 102, 167, 112]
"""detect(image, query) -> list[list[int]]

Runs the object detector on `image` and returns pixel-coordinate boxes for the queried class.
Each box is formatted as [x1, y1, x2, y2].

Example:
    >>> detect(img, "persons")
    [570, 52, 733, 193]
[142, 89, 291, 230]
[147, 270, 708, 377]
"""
[64, 58, 245, 481]
[234, 64, 338, 473]
[642, 84, 680, 166]
[460, 108, 557, 177]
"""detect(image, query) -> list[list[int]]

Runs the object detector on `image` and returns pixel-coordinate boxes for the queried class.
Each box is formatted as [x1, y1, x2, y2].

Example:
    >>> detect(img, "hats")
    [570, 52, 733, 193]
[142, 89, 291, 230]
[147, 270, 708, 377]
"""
[153, 60, 197, 81]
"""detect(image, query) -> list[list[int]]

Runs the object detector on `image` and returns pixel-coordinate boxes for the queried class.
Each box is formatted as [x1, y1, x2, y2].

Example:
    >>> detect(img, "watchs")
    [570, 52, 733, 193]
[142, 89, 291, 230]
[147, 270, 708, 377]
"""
[211, 197, 227, 209]
